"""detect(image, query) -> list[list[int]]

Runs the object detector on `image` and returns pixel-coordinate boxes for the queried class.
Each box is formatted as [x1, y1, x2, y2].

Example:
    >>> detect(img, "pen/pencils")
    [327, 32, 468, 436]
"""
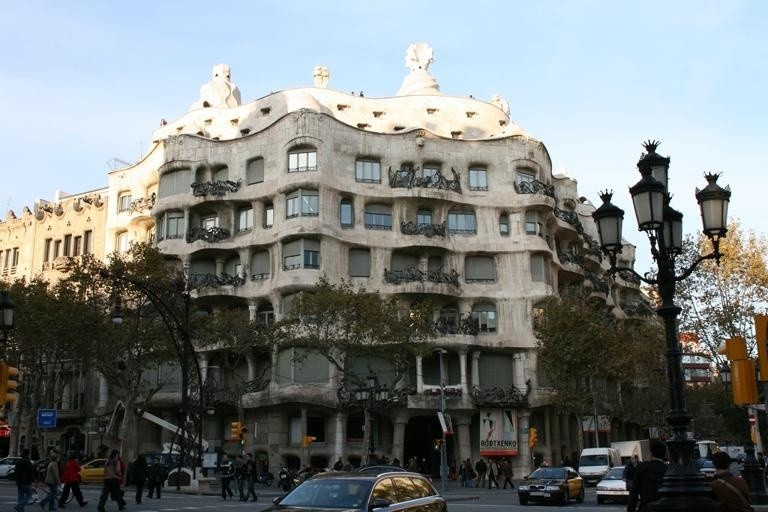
[125, 451, 208, 487]
[201, 447, 218, 477]
[576, 447, 622, 487]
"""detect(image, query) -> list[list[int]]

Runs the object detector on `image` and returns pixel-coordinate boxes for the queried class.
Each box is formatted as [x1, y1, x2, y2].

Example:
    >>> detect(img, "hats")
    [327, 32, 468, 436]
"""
[696, 458, 717, 481]
[518, 466, 584, 506]
[352, 465, 432, 484]
[0, 457, 35, 481]
[596, 465, 641, 504]
[6, 458, 66, 483]
[279, 467, 327, 489]
[61, 458, 109, 483]
[735, 453, 747, 465]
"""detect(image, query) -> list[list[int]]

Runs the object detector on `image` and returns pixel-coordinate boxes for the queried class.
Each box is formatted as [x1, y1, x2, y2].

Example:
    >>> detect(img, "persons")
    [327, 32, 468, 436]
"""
[545, 456, 556, 467]
[538, 455, 546, 466]
[626, 442, 668, 511]
[448, 461, 456, 481]
[14, 450, 168, 511]
[623, 450, 639, 508]
[643, 439, 756, 511]
[368, 448, 429, 475]
[343, 460, 352, 472]
[563, 455, 571, 464]
[233, 454, 246, 501]
[239, 453, 258, 501]
[460, 456, 516, 489]
[709, 450, 751, 504]
[218, 452, 236, 501]
[334, 456, 343, 471]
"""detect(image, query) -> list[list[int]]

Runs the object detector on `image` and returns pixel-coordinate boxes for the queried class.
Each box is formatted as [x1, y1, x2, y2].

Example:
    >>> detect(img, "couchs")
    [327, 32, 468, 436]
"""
[434, 440, 445, 446]
[241, 426, 248, 433]
[529, 428, 538, 448]
[715, 337, 727, 358]
[231, 422, 241, 440]
[238, 432, 248, 449]
[304, 436, 316, 448]
[0, 361, 19, 405]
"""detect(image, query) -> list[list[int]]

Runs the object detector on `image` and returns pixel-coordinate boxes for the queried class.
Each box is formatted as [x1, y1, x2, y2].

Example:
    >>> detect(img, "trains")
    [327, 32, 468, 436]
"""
[748, 414, 756, 426]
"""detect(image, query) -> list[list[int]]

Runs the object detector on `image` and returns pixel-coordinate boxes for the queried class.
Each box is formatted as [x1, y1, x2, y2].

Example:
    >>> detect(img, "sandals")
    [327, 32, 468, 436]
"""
[694, 439, 720, 460]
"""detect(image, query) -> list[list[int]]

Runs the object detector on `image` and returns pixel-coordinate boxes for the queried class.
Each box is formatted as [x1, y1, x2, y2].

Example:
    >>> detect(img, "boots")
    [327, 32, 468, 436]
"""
[259, 464, 447, 511]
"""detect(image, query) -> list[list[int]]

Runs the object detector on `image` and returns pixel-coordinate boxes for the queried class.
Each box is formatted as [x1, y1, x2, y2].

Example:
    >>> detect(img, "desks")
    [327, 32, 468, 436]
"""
[0, 289, 17, 361]
[591, 138, 731, 512]
[100, 268, 206, 491]
[719, 359, 768, 505]
[439, 349, 448, 491]
[352, 369, 389, 466]
[190, 372, 216, 480]
